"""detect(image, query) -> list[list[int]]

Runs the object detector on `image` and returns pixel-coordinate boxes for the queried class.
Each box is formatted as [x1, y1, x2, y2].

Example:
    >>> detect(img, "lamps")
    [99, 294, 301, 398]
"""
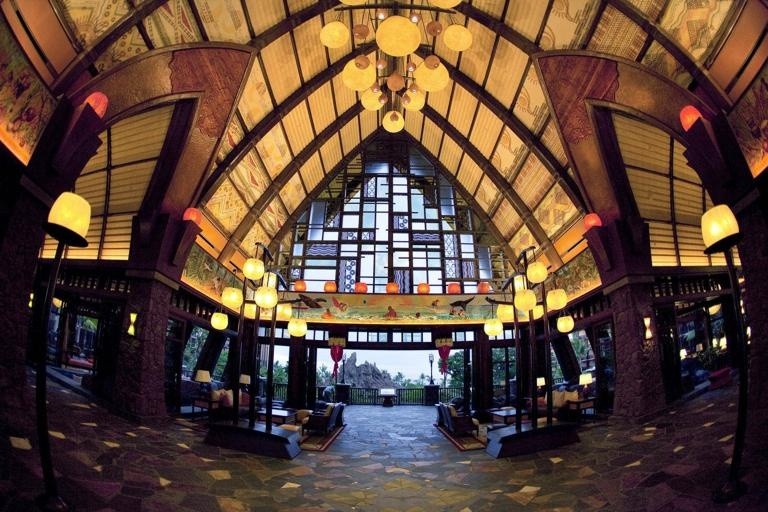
[239, 373, 251, 391]
[321, 0, 473, 135]
[536, 377, 546, 393]
[194, 370, 211, 400]
[578, 372, 593, 400]
[485, 246, 582, 459]
[203, 241, 308, 460]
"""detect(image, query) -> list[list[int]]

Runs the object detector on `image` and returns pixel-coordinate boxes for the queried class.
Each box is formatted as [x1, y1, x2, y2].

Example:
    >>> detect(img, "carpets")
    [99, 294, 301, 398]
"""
[433, 416, 590, 452]
[175, 416, 347, 451]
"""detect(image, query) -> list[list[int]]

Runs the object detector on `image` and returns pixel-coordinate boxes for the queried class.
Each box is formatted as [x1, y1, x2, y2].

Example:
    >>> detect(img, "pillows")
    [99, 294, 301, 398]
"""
[544, 389, 579, 408]
[212, 388, 242, 408]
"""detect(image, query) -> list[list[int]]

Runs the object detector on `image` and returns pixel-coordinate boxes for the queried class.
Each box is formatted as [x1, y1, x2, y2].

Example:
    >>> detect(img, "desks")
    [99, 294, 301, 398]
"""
[378, 395, 398, 407]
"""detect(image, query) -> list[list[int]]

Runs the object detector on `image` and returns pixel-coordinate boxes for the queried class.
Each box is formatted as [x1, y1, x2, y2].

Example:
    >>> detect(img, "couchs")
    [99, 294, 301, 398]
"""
[211, 392, 249, 413]
[526, 392, 586, 420]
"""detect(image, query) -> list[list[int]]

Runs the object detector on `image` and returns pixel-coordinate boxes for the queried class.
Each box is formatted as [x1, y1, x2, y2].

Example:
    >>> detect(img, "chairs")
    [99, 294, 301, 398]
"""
[302, 401, 342, 437]
[440, 402, 479, 440]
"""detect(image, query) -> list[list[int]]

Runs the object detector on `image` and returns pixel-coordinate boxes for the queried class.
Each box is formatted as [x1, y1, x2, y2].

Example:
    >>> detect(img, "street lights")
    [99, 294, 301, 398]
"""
[429, 353, 434, 384]
[205, 256, 308, 460]
[35, 189, 94, 511]
[341, 352, 346, 383]
[700, 202, 750, 503]
[483, 261, 581, 457]
